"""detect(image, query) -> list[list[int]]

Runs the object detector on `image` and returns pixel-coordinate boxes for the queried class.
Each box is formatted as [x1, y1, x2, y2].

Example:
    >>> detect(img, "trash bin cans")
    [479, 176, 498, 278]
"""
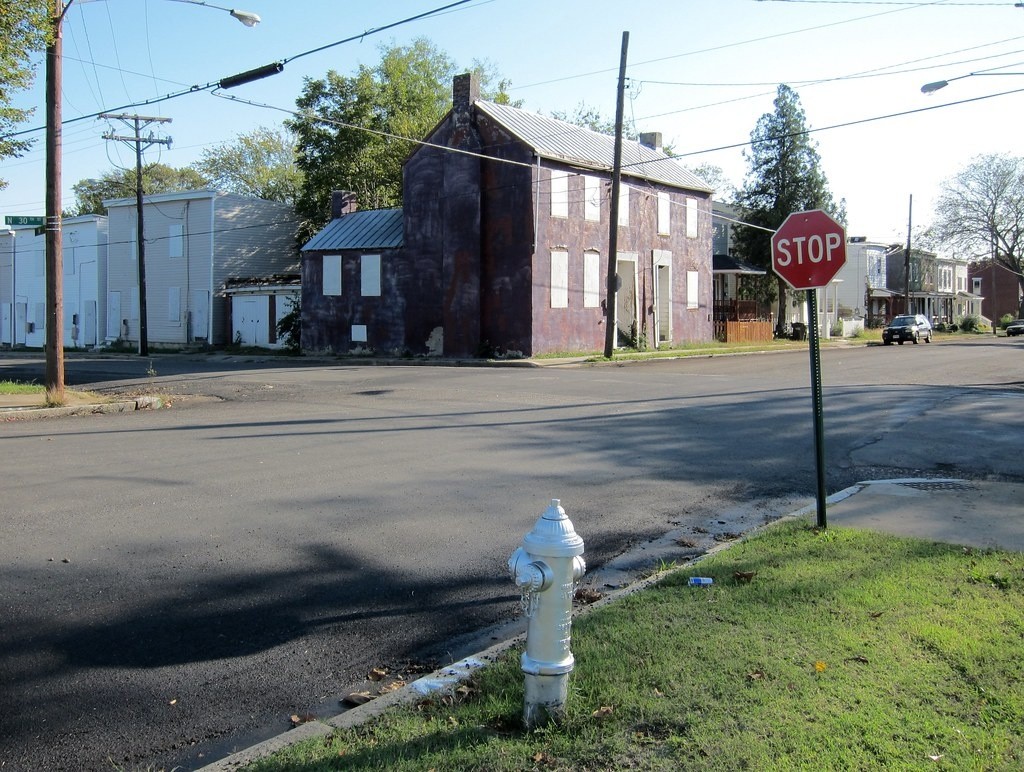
[791, 322, 805, 340]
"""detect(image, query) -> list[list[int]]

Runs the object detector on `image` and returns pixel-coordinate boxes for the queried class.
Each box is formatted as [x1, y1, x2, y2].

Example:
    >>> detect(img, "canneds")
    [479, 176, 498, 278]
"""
[688, 577, 713, 589]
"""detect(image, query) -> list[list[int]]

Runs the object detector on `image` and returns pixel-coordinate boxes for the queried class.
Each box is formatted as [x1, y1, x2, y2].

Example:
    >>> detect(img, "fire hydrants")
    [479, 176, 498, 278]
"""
[507, 499, 586, 725]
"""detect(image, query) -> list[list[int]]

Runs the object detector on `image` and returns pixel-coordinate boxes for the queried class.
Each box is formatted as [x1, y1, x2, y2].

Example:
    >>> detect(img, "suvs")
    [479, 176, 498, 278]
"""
[882, 313, 933, 344]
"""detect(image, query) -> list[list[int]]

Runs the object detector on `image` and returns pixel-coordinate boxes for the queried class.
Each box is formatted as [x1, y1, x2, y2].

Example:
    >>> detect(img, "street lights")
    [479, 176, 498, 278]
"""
[45, 0, 260, 405]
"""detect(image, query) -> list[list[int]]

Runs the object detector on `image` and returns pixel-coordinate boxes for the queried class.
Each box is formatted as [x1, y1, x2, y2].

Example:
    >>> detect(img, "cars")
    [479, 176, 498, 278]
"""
[1006, 319, 1024, 336]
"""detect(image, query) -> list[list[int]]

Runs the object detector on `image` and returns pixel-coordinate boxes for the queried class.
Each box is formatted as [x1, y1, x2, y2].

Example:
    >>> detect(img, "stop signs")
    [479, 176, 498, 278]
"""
[771, 209, 848, 291]
